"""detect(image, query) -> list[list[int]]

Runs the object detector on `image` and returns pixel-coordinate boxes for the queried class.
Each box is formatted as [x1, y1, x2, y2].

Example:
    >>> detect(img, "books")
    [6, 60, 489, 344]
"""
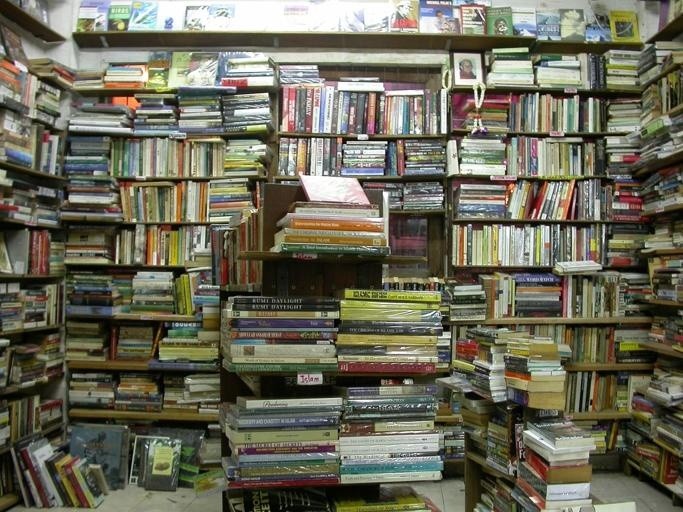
[0, 0, 683, 511]
